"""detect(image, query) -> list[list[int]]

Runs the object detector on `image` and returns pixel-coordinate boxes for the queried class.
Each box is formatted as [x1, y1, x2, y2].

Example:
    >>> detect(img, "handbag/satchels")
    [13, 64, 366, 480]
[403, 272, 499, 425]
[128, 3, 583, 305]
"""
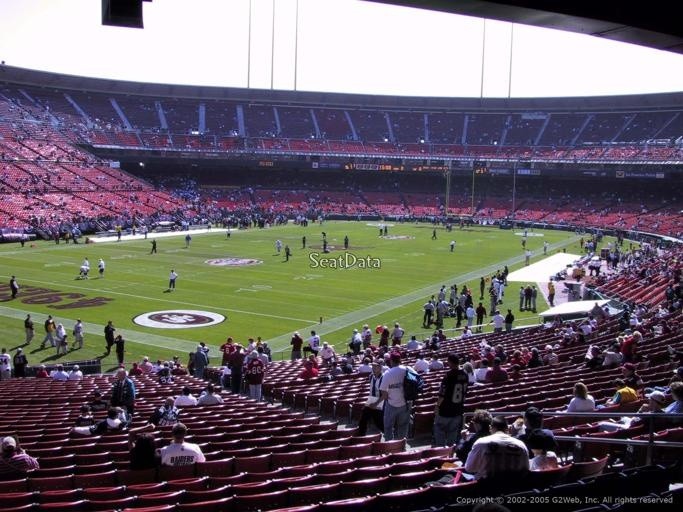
[423, 470, 472, 489]
[149, 408, 162, 425]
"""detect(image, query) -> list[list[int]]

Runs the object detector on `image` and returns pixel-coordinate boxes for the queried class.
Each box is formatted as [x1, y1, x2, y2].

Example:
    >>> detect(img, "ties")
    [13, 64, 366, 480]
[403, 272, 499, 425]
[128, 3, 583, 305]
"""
[119, 382, 123, 401]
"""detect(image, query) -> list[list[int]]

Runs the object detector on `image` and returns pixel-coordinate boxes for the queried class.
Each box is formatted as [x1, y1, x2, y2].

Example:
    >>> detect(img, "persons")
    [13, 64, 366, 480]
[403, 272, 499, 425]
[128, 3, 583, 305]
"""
[0, 261, 92, 479]
[92, 321, 421, 442]
[168, 270, 178, 290]
[0, 88, 682, 260]
[127, 432, 161, 469]
[160, 422, 206, 467]
[420, 260, 683, 512]
[97, 258, 104, 278]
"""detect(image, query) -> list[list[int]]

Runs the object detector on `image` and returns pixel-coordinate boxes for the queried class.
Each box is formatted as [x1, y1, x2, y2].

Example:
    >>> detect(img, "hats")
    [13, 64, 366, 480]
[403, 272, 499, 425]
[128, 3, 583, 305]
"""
[120, 356, 180, 368]
[248, 350, 260, 359]
[39, 364, 80, 371]
[171, 422, 189, 435]
[368, 362, 381, 367]
[390, 351, 401, 360]
[644, 390, 667, 404]
[624, 362, 636, 372]
[2, 436, 17, 451]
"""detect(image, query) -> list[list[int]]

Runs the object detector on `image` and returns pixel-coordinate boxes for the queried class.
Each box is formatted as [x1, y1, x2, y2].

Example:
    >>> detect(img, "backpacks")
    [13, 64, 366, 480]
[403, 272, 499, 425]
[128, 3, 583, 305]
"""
[403, 368, 424, 410]
[128, 436, 157, 472]
